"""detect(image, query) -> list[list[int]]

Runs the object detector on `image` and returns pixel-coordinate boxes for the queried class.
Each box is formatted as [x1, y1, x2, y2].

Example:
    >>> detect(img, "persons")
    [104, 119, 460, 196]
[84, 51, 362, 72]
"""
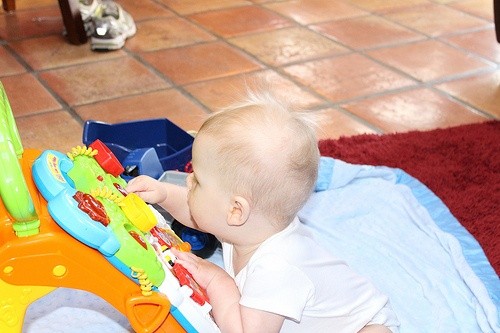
[125, 104, 402, 333]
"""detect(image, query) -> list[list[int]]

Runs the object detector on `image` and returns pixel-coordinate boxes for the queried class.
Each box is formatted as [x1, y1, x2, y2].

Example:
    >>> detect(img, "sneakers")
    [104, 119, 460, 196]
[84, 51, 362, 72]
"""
[91, 0, 137, 50]
[63, 0, 102, 37]
